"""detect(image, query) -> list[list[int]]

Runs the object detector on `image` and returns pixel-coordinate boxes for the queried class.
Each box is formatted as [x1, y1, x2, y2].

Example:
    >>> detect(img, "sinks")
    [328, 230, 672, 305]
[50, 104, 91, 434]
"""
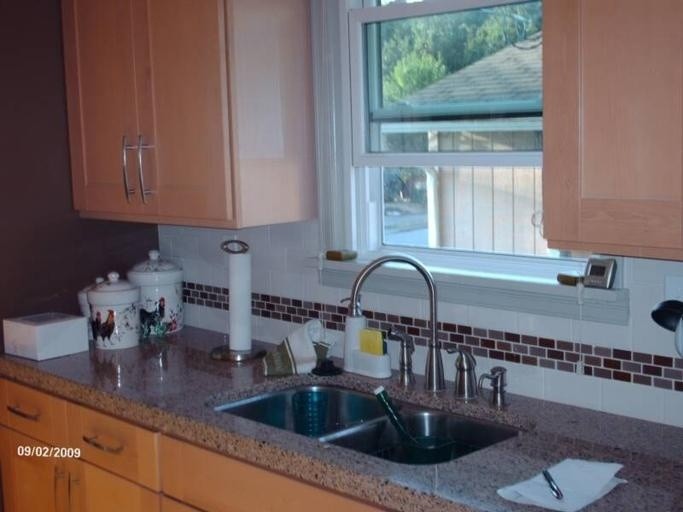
[206, 374, 403, 470]
[321, 406, 536, 467]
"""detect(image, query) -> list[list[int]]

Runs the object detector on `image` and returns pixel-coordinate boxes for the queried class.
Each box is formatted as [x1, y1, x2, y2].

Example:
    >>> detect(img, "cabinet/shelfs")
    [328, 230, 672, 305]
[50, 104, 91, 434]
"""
[542, 0, 683, 264]
[0, 374, 162, 512]
[60, 0, 320, 230]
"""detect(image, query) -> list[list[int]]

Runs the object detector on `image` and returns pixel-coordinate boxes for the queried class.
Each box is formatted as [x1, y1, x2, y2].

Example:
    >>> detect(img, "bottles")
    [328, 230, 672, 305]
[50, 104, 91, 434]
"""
[76, 248, 185, 350]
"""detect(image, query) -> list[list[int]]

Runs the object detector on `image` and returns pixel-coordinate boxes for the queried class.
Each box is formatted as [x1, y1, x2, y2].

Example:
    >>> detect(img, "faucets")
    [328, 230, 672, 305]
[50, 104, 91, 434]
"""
[350, 255, 445, 389]
[477, 367, 509, 407]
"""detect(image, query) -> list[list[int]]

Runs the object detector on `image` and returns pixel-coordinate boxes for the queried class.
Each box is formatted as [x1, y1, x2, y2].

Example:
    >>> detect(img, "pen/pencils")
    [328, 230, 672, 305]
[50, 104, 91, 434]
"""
[542, 468, 563, 500]
